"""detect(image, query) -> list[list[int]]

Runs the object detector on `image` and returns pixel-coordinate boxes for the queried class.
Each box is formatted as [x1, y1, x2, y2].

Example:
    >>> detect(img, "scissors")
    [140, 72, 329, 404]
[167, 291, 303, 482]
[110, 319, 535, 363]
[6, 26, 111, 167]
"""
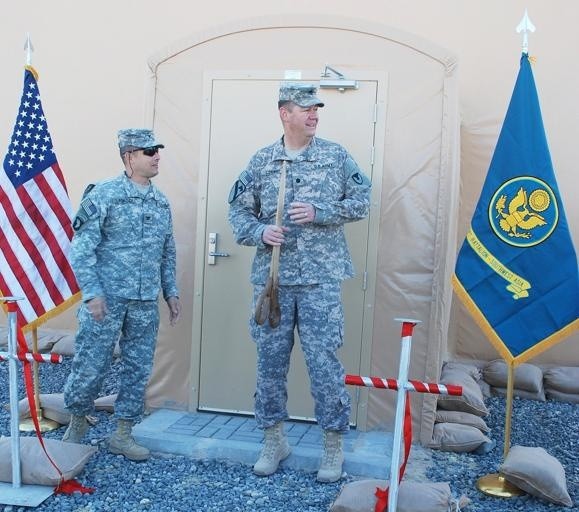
[254, 161, 286, 329]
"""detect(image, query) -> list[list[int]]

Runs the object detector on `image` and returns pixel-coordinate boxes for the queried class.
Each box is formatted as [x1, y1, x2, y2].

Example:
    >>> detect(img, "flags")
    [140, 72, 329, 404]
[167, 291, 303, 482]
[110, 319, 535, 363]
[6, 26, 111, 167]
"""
[1, 55, 86, 339]
[449, 45, 579, 369]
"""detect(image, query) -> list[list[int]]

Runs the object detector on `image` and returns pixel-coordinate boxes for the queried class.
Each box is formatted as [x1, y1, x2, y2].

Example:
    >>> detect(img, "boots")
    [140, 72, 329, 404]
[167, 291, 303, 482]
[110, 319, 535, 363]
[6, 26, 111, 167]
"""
[317, 430, 345, 483]
[62, 414, 87, 444]
[109, 420, 149, 461]
[253, 421, 291, 476]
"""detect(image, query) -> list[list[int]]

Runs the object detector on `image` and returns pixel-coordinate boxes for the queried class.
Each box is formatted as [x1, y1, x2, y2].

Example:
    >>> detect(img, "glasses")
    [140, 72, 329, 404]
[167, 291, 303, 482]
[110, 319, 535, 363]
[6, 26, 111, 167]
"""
[123, 146, 158, 156]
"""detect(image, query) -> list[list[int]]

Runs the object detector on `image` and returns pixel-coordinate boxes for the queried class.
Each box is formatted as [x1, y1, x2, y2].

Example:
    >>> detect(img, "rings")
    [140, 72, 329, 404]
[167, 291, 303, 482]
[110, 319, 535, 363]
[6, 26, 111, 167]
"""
[304, 212, 308, 218]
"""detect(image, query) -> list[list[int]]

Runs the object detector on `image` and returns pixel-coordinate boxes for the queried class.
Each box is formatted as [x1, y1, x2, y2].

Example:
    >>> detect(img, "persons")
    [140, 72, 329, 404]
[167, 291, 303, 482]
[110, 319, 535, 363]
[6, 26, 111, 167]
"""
[227, 82, 374, 485]
[58, 126, 183, 463]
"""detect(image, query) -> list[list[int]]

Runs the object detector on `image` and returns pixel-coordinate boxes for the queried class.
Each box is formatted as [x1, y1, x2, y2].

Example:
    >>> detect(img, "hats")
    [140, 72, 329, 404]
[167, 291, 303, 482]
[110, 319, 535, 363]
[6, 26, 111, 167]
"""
[279, 84, 324, 108]
[118, 128, 165, 148]
